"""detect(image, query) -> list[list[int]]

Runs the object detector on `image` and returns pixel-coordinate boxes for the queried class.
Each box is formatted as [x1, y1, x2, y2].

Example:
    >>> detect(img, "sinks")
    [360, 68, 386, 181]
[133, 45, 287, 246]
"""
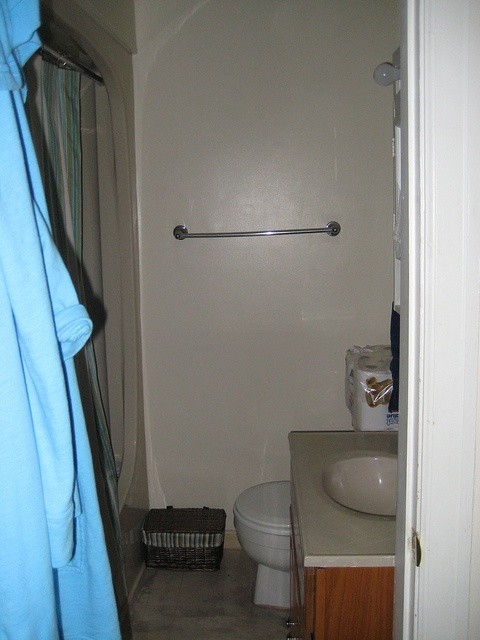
[288, 430, 398, 568]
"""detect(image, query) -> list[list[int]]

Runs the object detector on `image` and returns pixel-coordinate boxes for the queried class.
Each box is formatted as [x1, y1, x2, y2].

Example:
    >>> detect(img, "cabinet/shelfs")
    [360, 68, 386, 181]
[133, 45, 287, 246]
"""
[289, 491, 395, 640]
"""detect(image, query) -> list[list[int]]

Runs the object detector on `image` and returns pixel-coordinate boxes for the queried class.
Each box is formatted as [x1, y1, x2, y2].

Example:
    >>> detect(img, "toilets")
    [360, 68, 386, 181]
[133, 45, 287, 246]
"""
[232, 344, 394, 610]
